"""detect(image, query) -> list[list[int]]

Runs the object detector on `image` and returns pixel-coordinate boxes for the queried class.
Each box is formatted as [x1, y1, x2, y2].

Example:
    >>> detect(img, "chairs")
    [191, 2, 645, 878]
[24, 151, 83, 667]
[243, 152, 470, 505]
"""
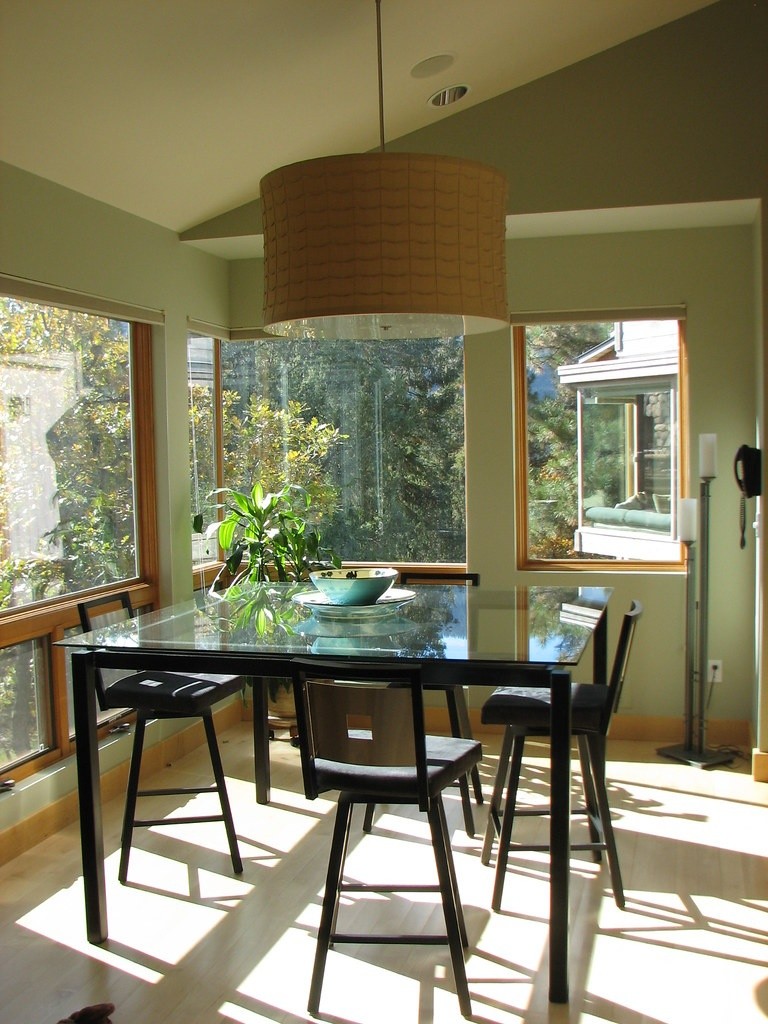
[290, 655, 483, 1015]
[363, 570, 485, 838]
[484, 599, 640, 910]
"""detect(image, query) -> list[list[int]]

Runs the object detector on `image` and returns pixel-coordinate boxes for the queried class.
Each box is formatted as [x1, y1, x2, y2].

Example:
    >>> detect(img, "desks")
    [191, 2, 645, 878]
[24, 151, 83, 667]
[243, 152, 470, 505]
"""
[53, 579, 613, 1003]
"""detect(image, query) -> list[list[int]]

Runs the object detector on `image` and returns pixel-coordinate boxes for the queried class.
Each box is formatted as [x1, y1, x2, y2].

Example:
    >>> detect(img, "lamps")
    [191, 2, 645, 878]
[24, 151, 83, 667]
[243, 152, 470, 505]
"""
[259, 1, 511, 343]
[658, 435, 737, 769]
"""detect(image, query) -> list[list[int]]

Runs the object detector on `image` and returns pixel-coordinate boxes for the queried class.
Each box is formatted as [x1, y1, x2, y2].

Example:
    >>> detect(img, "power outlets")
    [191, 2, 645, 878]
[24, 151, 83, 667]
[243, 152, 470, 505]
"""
[708, 659, 723, 686]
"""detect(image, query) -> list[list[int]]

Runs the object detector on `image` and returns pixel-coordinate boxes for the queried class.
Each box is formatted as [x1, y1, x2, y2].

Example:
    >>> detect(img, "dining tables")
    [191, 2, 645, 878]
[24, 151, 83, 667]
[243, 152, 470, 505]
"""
[76, 589, 245, 882]
